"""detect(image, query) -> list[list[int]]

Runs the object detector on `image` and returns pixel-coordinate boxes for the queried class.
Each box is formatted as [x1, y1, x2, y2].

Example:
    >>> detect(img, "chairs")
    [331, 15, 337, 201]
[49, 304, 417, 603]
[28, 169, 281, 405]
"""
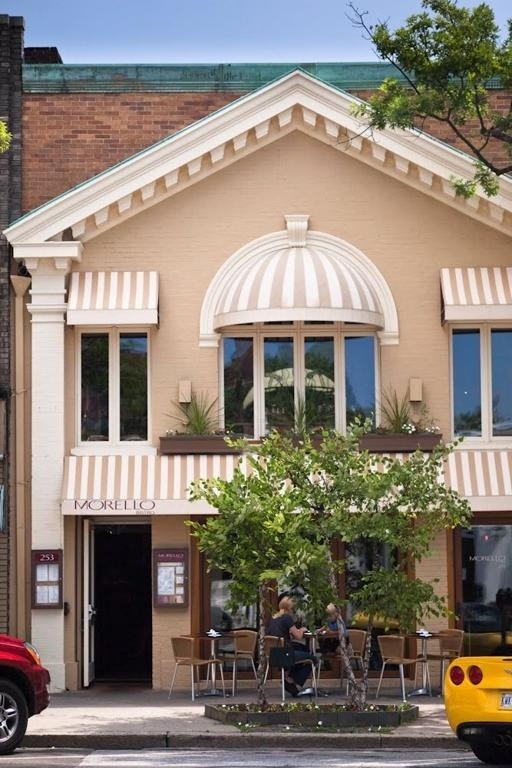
[167, 627, 466, 701]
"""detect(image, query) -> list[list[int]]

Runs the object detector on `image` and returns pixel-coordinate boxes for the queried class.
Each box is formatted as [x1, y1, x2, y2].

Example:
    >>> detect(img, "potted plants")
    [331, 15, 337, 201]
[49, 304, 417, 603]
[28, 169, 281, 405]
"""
[258, 375, 340, 454]
[157, 391, 244, 456]
[355, 383, 443, 453]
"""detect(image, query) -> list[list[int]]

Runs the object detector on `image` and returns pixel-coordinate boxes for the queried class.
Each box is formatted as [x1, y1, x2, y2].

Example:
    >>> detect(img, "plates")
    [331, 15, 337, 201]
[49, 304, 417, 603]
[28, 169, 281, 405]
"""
[416, 631, 432, 636]
[304, 633, 317, 635]
[206, 632, 222, 637]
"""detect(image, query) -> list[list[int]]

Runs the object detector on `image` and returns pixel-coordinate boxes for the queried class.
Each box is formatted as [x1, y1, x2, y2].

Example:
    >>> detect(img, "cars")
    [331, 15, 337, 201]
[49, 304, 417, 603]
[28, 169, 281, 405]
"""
[440, 652, 510, 764]
[2, 627, 52, 757]
[212, 577, 249, 624]
[352, 599, 510, 668]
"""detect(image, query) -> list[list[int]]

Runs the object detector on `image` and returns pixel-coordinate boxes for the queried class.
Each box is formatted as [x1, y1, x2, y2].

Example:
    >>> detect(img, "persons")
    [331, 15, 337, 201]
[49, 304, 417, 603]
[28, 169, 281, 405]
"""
[268, 595, 320, 699]
[316, 601, 351, 662]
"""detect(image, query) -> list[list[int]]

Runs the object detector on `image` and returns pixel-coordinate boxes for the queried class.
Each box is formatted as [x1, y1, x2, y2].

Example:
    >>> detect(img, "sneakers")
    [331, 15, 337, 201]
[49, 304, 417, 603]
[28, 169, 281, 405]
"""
[280, 679, 302, 697]
